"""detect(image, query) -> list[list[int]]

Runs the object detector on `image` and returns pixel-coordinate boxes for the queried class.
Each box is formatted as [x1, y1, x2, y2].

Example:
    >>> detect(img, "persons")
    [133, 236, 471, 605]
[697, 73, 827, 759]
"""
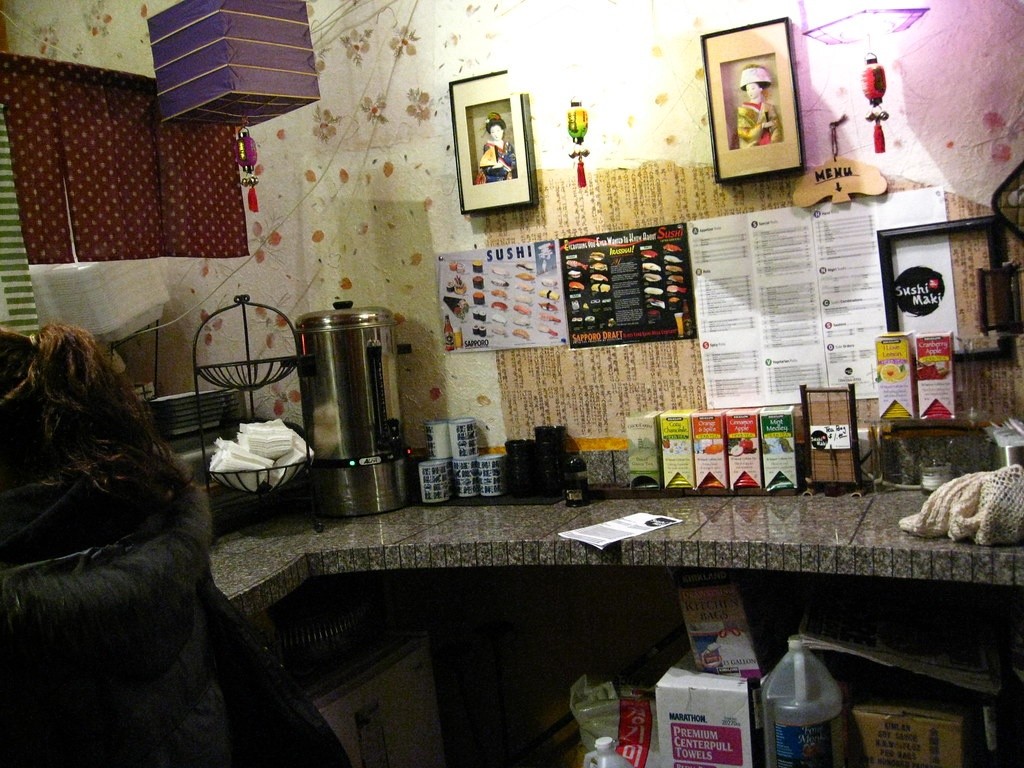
[474, 112, 517, 185]
[0, 324, 229, 768]
[737, 64, 783, 149]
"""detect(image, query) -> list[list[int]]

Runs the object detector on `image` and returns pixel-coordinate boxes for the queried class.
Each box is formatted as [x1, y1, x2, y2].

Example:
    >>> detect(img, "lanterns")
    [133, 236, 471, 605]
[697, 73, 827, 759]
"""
[235, 127, 260, 212]
[147, 0, 322, 126]
[799, 0, 930, 46]
[567, 96, 590, 187]
[862, 53, 889, 154]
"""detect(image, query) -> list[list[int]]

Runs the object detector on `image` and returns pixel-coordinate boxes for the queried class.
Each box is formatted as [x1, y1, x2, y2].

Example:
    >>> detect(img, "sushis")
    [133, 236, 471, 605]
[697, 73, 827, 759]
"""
[443, 260, 561, 340]
[566, 252, 611, 292]
[641, 243, 687, 309]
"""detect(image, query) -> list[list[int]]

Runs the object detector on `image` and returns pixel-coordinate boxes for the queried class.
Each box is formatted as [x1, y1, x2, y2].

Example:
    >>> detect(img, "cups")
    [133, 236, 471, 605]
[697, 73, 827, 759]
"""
[448, 419, 479, 459]
[418, 460, 451, 502]
[453, 327, 463, 347]
[424, 419, 451, 459]
[921, 461, 951, 491]
[674, 312, 684, 338]
[566, 457, 588, 506]
[453, 460, 478, 496]
[478, 455, 506, 496]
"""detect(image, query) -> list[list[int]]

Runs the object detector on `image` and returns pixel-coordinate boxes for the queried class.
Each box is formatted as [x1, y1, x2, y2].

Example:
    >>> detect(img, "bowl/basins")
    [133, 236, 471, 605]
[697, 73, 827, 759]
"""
[506, 423, 565, 496]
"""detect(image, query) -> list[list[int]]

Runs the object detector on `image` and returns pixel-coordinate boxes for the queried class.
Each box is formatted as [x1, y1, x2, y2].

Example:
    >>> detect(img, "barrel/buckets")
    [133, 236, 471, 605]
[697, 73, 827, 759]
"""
[989, 444, 1024, 471]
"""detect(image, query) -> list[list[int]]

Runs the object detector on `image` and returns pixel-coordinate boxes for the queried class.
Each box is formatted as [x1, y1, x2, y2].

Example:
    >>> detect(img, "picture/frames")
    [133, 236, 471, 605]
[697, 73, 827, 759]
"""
[877, 215, 1017, 364]
[449, 69, 539, 215]
[700, 17, 804, 184]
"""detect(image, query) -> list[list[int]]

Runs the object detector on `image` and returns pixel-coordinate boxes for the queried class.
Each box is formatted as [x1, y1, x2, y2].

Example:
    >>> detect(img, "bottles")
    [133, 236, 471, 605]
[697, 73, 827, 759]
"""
[583, 736, 637, 768]
[682, 300, 695, 338]
[763, 634, 847, 768]
[443, 315, 454, 351]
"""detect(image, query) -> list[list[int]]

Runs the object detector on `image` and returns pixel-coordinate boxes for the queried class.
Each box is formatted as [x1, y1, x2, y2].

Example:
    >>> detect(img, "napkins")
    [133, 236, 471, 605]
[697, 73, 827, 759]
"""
[210, 419, 314, 491]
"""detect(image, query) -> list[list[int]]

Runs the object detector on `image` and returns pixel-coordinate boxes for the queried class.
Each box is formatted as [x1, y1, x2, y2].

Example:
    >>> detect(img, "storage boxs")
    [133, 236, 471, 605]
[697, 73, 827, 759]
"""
[915, 330, 956, 420]
[875, 331, 915, 419]
[760, 405, 798, 491]
[725, 409, 762, 490]
[656, 650, 766, 768]
[667, 565, 810, 680]
[625, 410, 664, 490]
[691, 409, 729, 490]
[851, 666, 982, 768]
[661, 409, 696, 489]
